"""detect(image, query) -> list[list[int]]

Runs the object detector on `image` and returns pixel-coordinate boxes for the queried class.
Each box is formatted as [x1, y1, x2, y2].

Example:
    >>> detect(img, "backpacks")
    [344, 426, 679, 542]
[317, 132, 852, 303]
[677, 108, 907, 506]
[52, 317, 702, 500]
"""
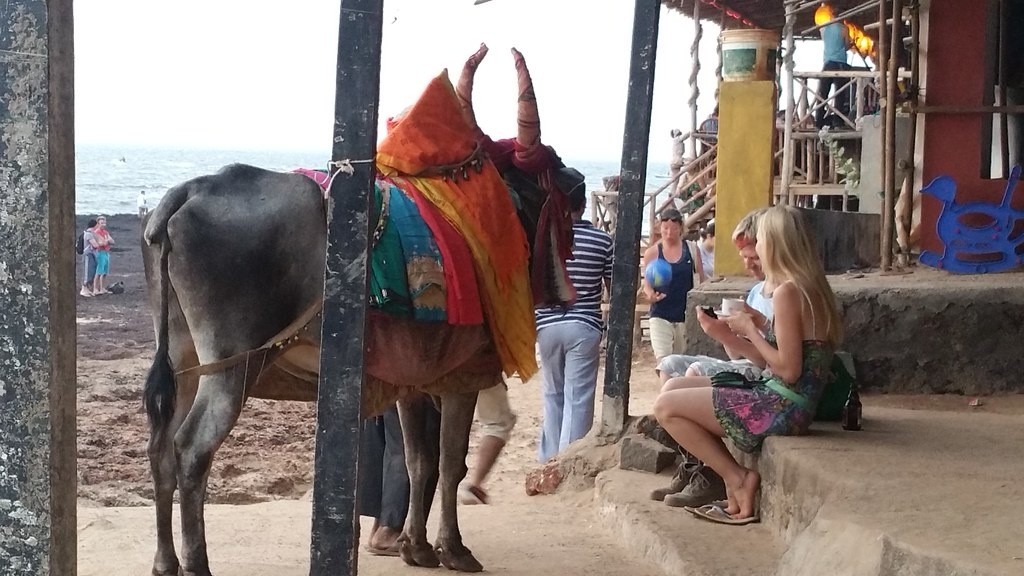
[76, 231, 92, 254]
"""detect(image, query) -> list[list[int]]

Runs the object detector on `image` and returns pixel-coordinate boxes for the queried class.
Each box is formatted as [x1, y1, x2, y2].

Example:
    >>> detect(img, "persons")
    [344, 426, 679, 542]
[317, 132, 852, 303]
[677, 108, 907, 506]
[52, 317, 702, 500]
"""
[643, 210, 716, 363]
[93, 216, 115, 295]
[652, 205, 843, 520]
[137, 190, 149, 217]
[534, 167, 615, 467]
[816, 9, 851, 128]
[460, 381, 517, 506]
[79, 220, 99, 298]
[357, 393, 442, 556]
[650, 208, 776, 508]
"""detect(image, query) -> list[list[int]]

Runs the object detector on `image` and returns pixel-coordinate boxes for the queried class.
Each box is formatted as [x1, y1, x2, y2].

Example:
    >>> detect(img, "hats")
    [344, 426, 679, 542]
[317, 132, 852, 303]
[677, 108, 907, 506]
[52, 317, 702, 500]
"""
[96, 216, 106, 221]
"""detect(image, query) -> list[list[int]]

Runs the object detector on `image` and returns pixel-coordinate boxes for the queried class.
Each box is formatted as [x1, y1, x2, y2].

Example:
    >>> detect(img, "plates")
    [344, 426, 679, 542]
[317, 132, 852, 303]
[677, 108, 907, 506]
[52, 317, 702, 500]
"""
[714, 310, 753, 318]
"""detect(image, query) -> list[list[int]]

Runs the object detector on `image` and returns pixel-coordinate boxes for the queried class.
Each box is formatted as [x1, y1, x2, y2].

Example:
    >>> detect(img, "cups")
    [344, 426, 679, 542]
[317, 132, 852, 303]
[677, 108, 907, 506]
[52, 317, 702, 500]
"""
[722, 297, 745, 315]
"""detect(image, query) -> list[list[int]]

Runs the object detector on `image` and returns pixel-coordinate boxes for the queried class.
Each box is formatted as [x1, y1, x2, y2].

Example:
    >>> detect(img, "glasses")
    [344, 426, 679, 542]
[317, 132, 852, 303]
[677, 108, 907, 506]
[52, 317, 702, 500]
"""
[662, 217, 680, 222]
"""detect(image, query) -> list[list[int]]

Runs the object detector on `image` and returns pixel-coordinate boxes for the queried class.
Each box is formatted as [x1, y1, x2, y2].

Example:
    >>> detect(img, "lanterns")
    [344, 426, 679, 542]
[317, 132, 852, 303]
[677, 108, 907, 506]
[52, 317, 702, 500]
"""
[815, 2, 877, 57]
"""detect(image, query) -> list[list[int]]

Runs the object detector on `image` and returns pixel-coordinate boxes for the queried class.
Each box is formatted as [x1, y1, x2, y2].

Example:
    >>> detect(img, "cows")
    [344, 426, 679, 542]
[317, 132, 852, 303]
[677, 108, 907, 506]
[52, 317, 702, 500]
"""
[138, 45, 576, 576]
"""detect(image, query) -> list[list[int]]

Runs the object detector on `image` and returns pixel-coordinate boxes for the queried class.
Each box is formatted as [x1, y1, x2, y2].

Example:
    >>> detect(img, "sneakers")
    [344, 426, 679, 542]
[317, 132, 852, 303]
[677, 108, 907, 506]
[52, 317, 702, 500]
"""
[666, 466, 727, 507]
[652, 462, 703, 501]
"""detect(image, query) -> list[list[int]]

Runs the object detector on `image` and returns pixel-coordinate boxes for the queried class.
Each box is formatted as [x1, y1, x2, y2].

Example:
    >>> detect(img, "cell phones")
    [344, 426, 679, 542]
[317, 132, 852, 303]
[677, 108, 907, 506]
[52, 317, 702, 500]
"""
[700, 305, 718, 319]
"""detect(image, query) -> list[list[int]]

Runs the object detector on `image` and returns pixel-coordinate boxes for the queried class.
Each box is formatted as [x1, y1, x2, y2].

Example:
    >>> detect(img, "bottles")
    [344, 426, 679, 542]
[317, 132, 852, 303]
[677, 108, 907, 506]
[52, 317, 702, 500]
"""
[842, 382, 862, 431]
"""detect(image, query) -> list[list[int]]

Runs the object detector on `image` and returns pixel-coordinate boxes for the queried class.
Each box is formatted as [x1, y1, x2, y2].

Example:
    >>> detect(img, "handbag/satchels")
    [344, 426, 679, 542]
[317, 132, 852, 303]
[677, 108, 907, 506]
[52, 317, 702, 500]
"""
[815, 351, 856, 421]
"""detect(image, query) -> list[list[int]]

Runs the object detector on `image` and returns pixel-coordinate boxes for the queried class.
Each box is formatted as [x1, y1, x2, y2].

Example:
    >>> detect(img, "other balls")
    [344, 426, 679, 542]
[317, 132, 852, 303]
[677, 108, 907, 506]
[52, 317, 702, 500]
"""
[645, 258, 673, 291]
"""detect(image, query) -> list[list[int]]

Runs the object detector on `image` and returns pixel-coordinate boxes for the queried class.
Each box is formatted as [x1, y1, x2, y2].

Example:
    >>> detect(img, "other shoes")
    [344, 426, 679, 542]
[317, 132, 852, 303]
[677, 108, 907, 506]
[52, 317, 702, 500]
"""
[100, 289, 113, 294]
[93, 291, 104, 295]
[80, 291, 95, 297]
[460, 485, 485, 504]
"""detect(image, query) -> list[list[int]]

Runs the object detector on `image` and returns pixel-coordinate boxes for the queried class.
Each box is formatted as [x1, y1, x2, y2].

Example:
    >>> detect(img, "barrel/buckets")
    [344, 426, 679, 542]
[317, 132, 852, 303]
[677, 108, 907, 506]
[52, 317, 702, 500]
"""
[721, 28, 781, 82]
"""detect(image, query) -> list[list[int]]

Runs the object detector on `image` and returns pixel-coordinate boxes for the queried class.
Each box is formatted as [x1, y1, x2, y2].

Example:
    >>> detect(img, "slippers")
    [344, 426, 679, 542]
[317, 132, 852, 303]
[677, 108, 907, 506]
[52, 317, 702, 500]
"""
[364, 536, 401, 555]
[685, 500, 759, 524]
